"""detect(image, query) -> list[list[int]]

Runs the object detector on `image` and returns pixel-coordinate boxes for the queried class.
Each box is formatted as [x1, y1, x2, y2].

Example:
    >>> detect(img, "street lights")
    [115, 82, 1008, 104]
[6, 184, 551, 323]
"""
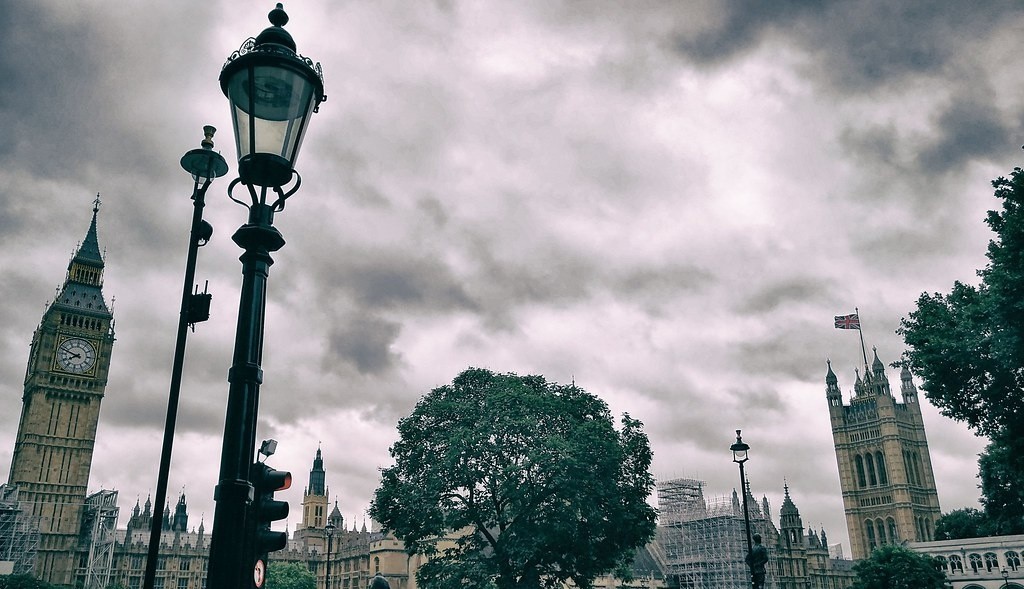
[142, 124, 226, 589]
[205, 1, 330, 589]
[729, 429, 760, 589]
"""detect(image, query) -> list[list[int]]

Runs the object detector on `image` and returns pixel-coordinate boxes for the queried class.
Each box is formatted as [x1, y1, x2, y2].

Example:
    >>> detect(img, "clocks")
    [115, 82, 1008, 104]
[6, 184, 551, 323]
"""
[27, 340, 39, 374]
[56, 337, 97, 373]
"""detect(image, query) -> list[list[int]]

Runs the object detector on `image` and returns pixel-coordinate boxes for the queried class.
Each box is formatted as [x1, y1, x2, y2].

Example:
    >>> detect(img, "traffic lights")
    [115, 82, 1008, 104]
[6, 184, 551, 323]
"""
[249, 462, 292, 554]
